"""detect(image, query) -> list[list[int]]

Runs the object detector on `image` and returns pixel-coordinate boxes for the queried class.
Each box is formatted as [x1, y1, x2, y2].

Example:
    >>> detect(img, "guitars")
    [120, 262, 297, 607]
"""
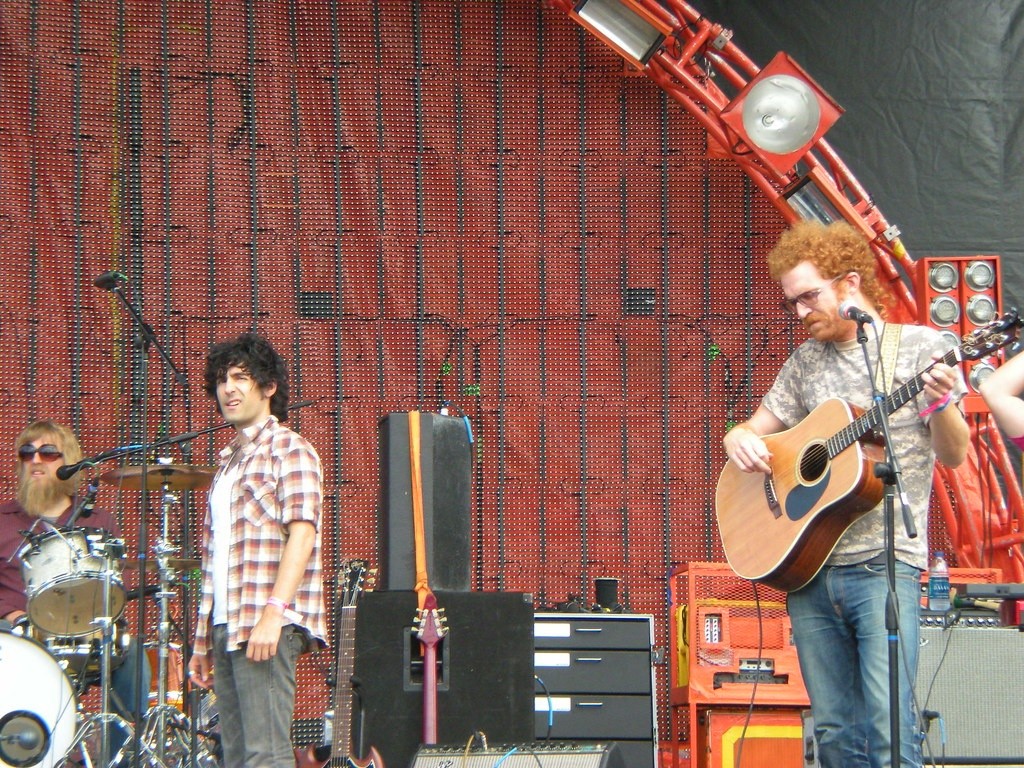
[716, 305, 1024, 593]
[300, 556, 384, 768]
[411, 592, 450, 744]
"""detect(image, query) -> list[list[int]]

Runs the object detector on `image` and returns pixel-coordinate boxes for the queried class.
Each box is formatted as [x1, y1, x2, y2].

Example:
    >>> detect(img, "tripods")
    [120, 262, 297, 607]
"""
[53, 471, 220, 768]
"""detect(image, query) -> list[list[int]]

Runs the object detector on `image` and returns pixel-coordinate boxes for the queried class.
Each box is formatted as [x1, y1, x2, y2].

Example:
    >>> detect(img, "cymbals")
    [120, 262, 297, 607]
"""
[119, 558, 202, 570]
[101, 464, 220, 492]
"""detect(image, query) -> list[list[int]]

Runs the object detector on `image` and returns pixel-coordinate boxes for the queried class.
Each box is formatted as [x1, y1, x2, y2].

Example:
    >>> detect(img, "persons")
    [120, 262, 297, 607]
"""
[978, 349, 1024, 458]
[723, 213, 971, 767]
[186, 331, 331, 768]
[0, 419, 151, 768]
[927, 390, 953, 411]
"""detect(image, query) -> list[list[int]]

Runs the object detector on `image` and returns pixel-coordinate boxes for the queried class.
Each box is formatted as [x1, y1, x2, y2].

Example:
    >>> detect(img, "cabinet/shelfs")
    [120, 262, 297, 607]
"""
[534, 612, 659, 768]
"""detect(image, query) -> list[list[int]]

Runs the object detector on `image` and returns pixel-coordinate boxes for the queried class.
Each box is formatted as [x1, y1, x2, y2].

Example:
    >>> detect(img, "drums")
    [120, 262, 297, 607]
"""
[18, 525, 127, 637]
[0, 618, 83, 768]
[13, 613, 131, 683]
[142, 642, 194, 701]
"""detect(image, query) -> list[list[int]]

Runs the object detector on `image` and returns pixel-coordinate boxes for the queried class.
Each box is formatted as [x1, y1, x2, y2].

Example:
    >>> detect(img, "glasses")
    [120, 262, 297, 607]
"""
[18, 443, 64, 463]
[781, 272, 848, 314]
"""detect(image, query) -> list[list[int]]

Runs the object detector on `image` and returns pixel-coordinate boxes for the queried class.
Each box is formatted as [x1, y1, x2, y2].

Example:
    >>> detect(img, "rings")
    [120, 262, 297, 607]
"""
[932, 383, 938, 388]
[189, 671, 198, 674]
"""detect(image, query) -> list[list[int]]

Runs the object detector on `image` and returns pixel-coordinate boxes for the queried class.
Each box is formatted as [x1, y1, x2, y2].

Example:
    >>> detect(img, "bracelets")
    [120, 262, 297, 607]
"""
[267, 597, 288, 611]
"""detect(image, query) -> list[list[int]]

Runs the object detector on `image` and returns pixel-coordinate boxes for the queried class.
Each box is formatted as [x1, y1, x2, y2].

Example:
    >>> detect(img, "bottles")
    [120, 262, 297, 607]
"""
[927, 552, 951, 611]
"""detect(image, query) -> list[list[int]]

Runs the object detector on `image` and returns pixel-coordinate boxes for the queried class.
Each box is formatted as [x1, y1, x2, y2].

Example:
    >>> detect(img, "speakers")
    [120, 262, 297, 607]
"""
[350, 588, 536, 768]
[377, 410, 474, 591]
[914, 626, 1024, 765]
[409, 741, 627, 768]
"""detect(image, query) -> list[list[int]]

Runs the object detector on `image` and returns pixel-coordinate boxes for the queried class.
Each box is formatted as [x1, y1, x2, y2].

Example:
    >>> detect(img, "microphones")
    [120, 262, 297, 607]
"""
[18, 726, 42, 750]
[80, 478, 99, 518]
[93, 271, 122, 289]
[919, 709, 940, 719]
[839, 302, 873, 324]
[56, 449, 114, 480]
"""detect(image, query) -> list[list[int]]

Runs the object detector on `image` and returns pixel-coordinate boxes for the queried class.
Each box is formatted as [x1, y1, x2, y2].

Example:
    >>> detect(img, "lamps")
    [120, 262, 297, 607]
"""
[721, 53, 845, 178]
[569, 0, 671, 73]
[916, 257, 1004, 413]
